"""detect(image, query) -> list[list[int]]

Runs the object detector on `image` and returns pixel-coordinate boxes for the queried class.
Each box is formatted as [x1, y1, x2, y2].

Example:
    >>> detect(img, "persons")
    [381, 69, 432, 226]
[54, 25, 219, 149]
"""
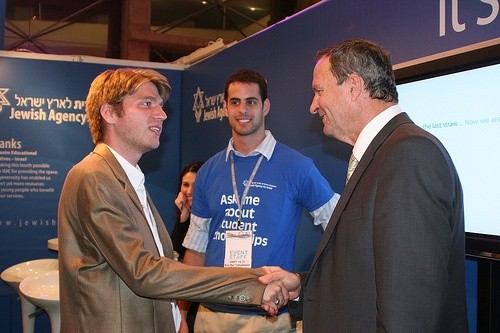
[258, 38, 469, 333]
[57, 68, 289, 333]
[177, 68, 339, 333]
[170, 161, 200, 333]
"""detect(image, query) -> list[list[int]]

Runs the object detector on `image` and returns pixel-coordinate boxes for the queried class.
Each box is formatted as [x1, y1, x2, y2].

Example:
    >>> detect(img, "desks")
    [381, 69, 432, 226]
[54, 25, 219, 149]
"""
[47, 238, 58, 251]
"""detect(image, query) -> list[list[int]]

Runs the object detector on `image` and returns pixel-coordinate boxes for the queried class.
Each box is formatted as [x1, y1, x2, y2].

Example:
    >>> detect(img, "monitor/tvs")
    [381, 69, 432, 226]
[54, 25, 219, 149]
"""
[465, 231, 500, 333]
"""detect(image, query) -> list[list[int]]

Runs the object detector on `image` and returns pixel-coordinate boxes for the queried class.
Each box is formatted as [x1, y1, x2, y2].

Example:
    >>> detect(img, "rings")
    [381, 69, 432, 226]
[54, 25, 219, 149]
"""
[276, 299, 279, 305]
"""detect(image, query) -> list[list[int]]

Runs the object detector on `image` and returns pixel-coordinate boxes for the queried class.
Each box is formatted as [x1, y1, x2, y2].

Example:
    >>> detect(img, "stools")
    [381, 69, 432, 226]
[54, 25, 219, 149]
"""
[0, 258, 58, 332]
[19, 272, 60, 333]
